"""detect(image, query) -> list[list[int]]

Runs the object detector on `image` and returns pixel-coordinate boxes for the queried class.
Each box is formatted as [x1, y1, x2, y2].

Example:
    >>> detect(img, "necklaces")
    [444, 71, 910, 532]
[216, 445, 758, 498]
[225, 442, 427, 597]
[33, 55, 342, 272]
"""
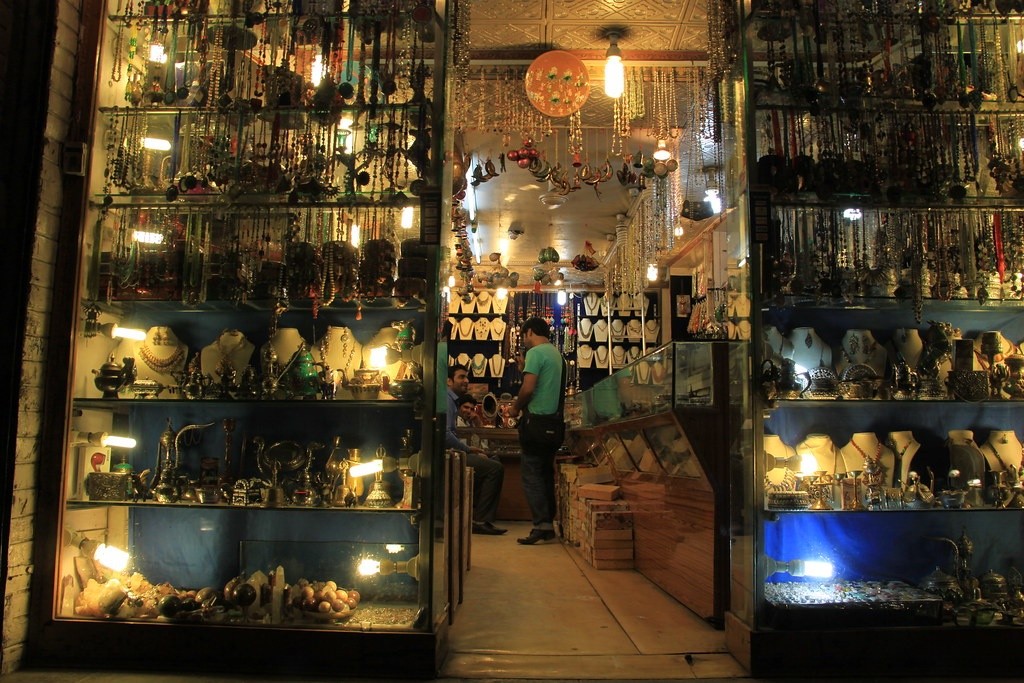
[84, 0, 1024, 497]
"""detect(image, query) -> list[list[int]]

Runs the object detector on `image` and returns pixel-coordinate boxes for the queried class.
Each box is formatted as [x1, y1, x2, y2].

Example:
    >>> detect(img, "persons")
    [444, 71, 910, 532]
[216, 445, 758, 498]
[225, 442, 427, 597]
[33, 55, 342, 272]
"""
[454, 394, 484, 457]
[445, 364, 507, 535]
[506, 317, 568, 543]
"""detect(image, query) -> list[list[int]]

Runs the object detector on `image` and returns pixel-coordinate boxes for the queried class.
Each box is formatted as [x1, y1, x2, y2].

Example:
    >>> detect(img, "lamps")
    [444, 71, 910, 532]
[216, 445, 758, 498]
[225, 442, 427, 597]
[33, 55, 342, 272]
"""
[348, 450, 420, 478]
[113, 36, 164, 65]
[357, 554, 419, 578]
[557, 279, 567, 304]
[704, 167, 722, 214]
[539, 127, 569, 209]
[67, 531, 129, 572]
[68, 429, 135, 450]
[368, 343, 423, 368]
[85, 321, 143, 344]
[117, 225, 165, 248]
[603, 31, 624, 99]
[103, 129, 169, 153]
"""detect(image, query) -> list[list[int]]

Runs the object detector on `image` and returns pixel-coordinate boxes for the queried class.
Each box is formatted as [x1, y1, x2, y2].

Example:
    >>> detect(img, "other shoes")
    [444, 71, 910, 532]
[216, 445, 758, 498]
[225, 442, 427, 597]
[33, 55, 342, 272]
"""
[472, 521, 508, 535]
[518, 529, 556, 544]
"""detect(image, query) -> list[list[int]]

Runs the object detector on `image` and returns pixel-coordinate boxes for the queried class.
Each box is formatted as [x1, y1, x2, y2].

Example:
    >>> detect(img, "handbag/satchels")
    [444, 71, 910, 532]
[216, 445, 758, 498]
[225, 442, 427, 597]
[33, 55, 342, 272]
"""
[518, 413, 566, 454]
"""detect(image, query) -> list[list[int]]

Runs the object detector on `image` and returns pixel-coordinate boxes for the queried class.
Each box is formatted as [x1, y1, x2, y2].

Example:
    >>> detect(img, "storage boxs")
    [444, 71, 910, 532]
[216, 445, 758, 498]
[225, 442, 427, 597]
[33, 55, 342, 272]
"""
[555, 464, 633, 570]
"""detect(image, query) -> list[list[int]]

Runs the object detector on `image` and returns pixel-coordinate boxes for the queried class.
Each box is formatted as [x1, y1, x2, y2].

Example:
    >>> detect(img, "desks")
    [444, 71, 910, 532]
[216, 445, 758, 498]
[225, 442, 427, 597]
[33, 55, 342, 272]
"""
[454, 427, 521, 458]
